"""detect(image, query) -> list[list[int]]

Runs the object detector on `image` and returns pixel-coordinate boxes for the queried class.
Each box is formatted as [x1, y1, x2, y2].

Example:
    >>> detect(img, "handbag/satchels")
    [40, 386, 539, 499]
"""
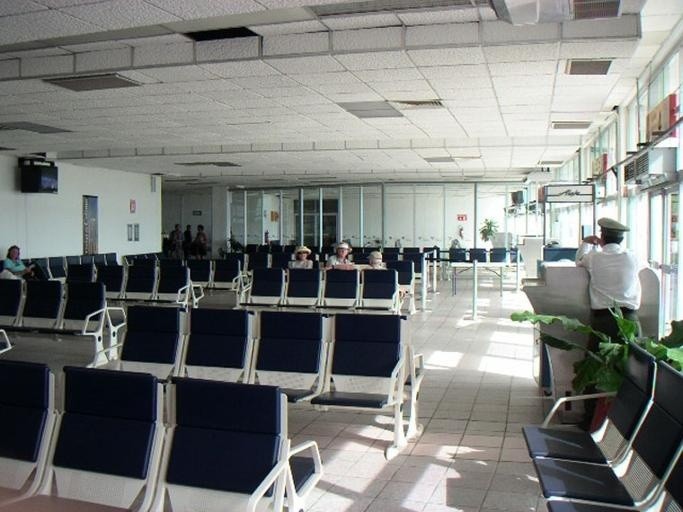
[28, 260, 49, 281]
[0, 268, 26, 285]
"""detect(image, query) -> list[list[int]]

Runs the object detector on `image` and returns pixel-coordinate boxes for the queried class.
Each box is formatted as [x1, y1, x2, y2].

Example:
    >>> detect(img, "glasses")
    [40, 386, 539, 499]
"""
[297, 252, 309, 255]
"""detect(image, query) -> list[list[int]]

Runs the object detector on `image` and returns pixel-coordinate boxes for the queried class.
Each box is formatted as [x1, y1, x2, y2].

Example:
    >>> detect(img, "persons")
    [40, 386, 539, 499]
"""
[367, 251, 408, 315]
[193, 224, 207, 260]
[169, 222, 183, 259]
[288, 245, 314, 270]
[325, 242, 355, 272]
[575, 217, 642, 433]
[3, 245, 36, 282]
[183, 224, 193, 259]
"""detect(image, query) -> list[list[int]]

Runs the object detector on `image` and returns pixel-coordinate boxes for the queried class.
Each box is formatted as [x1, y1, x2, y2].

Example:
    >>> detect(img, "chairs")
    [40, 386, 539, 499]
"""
[224, 252, 317, 268]
[353, 270, 398, 313]
[94, 266, 123, 298]
[131, 259, 181, 268]
[94, 306, 185, 377]
[1, 366, 164, 511]
[244, 244, 310, 253]
[405, 345, 424, 439]
[59, 283, 108, 369]
[186, 259, 209, 288]
[319, 270, 360, 309]
[287, 439, 322, 512]
[520, 340, 655, 467]
[177, 308, 253, 385]
[403, 247, 419, 261]
[489, 248, 506, 262]
[156, 266, 189, 313]
[66, 253, 118, 265]
[311, 313, 409, 462]
[469, 248, 485, 263]
[0, 329, 16, 355]
[422, 247, 440, 267]
[240, 269, 285, 304]
[543, 451, 682, 512]
[189, 281, 204, 310]
[405, 253, 426, 311]
[0, 279, 23, 327]
[66, 265, 91, 285]
[207, 259, 239, 310]
[387, 261, 420, 315]
[0, 359, 56, 509]
[282, 269, 323, 306]
[520, 360, 682, 511]
[449, 248, 465, 262]
[0, 257, 47, 280]
[147, 376, 288, 511]
[16, 281, 62, 329]
[246, 311, 326, 404]
[122, 266, 155, 301]
[337, 248, 397, 263]
[121, 253, 180, 262]
[105, 306, 127, 363]
[48, 257, 67, 278]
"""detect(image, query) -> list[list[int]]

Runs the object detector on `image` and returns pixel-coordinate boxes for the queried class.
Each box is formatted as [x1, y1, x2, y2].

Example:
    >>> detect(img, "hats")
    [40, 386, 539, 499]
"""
[336, 242, 353, 253]
[295, 246, 312, 256]
[598, 218, 630, 237]
[366, 250, 382, 259]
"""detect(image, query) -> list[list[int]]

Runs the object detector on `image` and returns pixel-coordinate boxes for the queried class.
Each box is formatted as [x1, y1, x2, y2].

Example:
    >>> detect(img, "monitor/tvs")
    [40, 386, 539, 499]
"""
[21, 165, 58, 194]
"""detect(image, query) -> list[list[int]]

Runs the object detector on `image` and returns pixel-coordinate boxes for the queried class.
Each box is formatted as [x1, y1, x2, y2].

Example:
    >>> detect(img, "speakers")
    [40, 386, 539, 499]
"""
[151, 176, 156, 192]
